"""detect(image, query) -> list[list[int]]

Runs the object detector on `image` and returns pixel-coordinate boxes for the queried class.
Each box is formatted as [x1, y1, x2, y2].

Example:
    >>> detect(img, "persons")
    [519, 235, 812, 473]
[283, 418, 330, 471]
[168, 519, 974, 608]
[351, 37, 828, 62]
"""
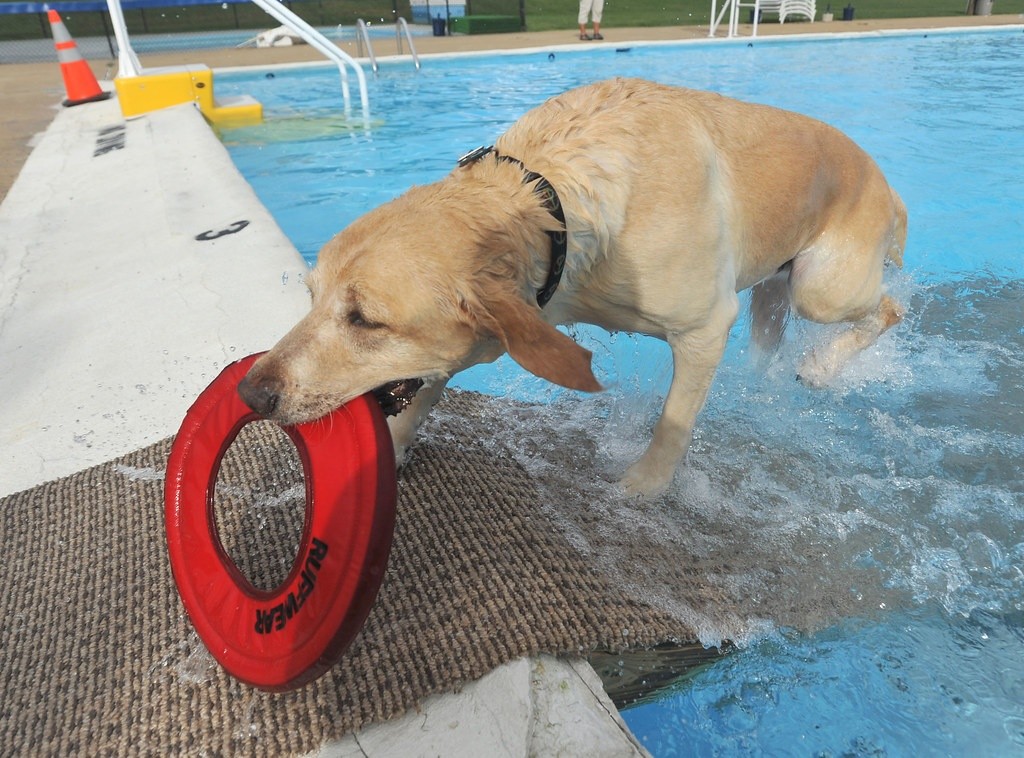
[578, 0, 605, 41]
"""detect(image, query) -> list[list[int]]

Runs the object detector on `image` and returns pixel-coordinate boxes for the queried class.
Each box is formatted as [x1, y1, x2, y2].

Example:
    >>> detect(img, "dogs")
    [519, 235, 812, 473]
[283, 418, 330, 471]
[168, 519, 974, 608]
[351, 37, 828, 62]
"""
[236, 76, 913, 496]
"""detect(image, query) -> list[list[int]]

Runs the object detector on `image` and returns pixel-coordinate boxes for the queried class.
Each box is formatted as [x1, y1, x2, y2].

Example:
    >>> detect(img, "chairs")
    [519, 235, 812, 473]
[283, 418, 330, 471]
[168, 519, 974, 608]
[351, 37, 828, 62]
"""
[709, 0, 816, 38]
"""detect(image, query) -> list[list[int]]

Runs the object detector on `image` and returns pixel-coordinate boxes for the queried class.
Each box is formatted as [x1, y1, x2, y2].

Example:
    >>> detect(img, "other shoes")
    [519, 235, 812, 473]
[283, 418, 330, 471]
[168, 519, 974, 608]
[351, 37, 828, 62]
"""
[580, 35, 592, 40]
[593, 33, 603, 40]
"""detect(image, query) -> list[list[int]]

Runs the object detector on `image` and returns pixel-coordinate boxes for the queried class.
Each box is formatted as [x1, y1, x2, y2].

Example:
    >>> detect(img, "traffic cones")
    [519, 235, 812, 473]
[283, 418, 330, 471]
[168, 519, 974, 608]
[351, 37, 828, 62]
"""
[48, 9, 111, 108]
